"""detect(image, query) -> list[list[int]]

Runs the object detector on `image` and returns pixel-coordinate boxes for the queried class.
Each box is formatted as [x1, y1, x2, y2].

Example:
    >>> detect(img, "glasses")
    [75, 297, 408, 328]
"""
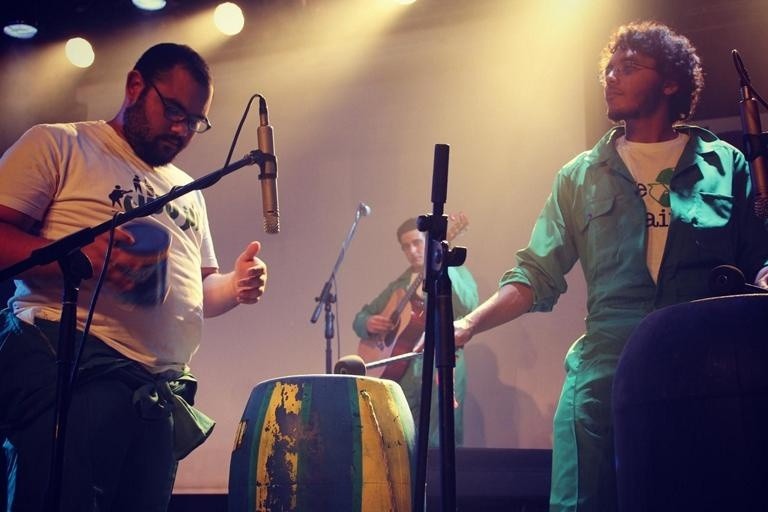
[145, 79, 211, 133]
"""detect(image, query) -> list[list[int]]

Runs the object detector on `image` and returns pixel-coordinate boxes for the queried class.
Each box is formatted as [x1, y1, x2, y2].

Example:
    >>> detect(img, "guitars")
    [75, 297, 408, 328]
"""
[357, 212, 470, 383]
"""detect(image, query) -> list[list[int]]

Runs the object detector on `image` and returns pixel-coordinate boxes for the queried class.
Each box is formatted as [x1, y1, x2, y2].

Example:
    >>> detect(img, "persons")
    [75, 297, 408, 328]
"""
[1, 42, 268, 512]
[412, 19, 766, 512]
[346, 209, 481, 447]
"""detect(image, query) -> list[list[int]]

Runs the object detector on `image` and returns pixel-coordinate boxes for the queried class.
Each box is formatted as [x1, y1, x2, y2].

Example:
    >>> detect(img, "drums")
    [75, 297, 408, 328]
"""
[612, 293, 768, 511]
[228, 374, 426, 512]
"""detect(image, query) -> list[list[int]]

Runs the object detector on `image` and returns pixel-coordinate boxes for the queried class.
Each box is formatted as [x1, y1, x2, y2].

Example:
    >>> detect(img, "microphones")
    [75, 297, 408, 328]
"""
[739, 80, 768, 219]
[259, 95, 280, 233]
[360, 202, 371, 216]
